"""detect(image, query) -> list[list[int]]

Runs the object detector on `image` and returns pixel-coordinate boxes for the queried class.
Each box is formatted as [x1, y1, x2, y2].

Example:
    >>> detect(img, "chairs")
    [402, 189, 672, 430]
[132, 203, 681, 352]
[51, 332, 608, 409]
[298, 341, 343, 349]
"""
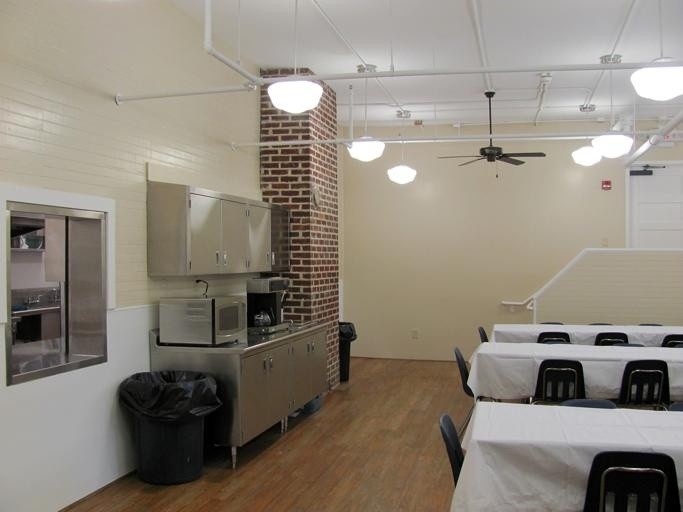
[544, 341, 571, 344]
[537, 332, 570, 344]
[595, 332, 628, 346]
[478, 326, 488, 342]
[582, 450, 681, 512]
[559, 398, 616, 409]
[529, 359, 586, 402]
[663, 401, 683, 412]
[613, 343, 644, 347]
[438, 413, 464, 486]
[638, 323, 662, 326]
[589, 322, 613, 325]
[674, 344, 683, 348]
[619, 359, 669, 409]
[454, 346, 501, 439]
[661, 334, 683, 348]
[540, 321, 563, 325]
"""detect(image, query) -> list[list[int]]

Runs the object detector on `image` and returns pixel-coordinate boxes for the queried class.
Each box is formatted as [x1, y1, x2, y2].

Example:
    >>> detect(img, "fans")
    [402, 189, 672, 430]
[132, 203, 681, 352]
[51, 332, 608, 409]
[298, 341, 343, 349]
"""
[434, 90, 547, 168]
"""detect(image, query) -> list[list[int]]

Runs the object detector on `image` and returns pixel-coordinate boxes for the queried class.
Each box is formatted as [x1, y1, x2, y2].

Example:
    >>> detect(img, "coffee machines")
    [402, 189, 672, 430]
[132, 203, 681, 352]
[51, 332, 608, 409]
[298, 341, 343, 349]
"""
[247, 276, 292, 334]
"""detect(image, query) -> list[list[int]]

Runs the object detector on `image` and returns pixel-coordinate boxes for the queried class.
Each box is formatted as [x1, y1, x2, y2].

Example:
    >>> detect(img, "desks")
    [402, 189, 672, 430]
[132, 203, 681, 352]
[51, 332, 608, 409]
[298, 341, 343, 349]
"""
[449, 401, 683, 512]
[491, 323, 683, 347]
[465, 342, 683, 405]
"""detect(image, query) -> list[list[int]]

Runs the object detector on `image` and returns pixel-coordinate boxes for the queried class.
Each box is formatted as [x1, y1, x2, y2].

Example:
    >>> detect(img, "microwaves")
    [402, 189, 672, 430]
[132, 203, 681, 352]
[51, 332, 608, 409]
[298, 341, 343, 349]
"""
[159, 295, 247, 345]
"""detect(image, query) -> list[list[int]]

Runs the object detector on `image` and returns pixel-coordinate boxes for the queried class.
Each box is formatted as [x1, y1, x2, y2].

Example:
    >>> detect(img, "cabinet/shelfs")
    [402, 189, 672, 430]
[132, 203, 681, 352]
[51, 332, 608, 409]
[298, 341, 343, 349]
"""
[290, 329, 330, 419]
[238, 343, 293, 446]
[146, 179, 248, 277]
[249, 198, 291, 273]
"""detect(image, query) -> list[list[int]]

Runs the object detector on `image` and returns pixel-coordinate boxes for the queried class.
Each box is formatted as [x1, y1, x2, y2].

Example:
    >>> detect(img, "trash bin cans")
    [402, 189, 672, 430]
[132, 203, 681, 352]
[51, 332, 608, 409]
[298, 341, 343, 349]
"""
[339, 321, 357, 382]
[118, 371, 221, 485]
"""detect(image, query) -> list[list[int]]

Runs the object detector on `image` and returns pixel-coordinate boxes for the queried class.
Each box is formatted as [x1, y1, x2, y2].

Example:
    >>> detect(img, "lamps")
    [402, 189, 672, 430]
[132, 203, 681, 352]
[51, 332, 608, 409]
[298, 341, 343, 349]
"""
[571, 105, 602, 167]
[347, 64, 386, 164]
[267, 0, 323, 115]
[387, 110, 417, 186]
[630, 0, 683, 102]
[591, 54, 634, 160]
